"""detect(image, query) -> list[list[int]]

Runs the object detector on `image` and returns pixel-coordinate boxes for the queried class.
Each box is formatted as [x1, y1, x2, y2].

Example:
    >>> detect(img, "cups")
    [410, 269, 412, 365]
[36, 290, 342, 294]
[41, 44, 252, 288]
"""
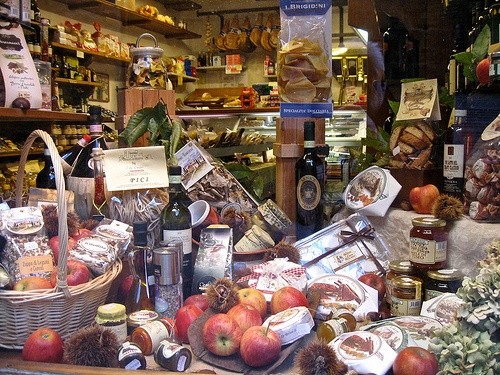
[188, 200, 222, 243]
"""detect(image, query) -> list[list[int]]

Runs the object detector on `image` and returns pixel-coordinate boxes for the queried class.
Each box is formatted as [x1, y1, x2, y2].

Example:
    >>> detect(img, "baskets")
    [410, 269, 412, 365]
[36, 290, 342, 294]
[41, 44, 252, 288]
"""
[0, 130, 122, 349]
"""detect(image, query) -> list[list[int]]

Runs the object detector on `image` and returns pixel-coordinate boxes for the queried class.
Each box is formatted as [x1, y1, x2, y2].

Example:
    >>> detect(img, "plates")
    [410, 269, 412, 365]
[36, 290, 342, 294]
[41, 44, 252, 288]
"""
[5, 214, 44, 235]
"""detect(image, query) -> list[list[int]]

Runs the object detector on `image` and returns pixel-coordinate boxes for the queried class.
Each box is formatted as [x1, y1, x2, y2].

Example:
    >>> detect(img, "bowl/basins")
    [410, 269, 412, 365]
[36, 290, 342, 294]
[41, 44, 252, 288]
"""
[232, 235, 286, 278]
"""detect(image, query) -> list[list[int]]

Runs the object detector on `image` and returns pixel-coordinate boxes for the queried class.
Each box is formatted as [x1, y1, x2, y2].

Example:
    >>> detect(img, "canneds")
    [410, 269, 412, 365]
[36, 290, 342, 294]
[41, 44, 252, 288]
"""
[383, 217, 459, 317]
[94, 303, 192, 373]
[486, 52, 500, 81]
[50, 124, 88, 146]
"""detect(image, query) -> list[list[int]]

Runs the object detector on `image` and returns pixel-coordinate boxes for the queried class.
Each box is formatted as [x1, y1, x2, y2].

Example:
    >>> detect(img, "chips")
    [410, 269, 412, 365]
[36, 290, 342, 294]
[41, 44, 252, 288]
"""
[277, 36, 331, 103]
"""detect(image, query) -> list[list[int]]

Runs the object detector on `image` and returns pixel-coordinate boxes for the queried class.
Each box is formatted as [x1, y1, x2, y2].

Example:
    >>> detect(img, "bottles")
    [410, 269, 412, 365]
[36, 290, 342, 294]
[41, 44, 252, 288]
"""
[197, 49, 213, 67]
[36, 126, 107, 193]
[438, 110, 472, 204]
[28, 42, 97, 82]
[150, 240, 183, 321]
[3, 184, 12, 209]
[161, 165, 193, 288]
[488, 52, 500, 79]
[294, 120, 325, 241]
[122, 221, 155, 336]
[268, 63, 274, 75]
[31, 0, 41, 22]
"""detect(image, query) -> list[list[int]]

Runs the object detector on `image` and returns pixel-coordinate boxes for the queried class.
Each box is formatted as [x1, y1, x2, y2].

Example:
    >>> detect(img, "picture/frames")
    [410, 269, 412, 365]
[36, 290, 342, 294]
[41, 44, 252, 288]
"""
[88, 72, 110, 102]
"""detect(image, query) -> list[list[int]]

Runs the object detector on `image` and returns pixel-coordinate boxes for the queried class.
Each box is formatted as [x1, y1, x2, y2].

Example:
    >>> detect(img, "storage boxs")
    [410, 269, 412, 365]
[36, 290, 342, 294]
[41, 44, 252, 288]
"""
[166, 141, 260, 217]
[293, 211, 394, 281]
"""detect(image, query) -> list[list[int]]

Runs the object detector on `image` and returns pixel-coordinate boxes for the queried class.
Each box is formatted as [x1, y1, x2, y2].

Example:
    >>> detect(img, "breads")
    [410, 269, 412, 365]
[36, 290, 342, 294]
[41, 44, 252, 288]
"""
[389, 120, 435, 168]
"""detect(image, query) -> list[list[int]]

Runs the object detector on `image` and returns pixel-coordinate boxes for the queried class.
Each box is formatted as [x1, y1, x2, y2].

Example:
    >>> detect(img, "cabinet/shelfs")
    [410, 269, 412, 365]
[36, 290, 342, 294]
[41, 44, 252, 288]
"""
[175, 105, 367, 205]
[0, 0, 201, 157]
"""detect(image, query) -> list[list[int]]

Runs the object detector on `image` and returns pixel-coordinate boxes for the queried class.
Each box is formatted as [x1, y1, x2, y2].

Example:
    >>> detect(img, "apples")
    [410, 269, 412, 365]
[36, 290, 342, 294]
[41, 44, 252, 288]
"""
[393, 347, 439, 375]
[10, 229, 92, 292]
[476, 59, 491, 84]
[176, 287, 310, 368]
[358, 273, 392, 319]
[22, 329, 65, 363]
[409, 184, 440, 214]
